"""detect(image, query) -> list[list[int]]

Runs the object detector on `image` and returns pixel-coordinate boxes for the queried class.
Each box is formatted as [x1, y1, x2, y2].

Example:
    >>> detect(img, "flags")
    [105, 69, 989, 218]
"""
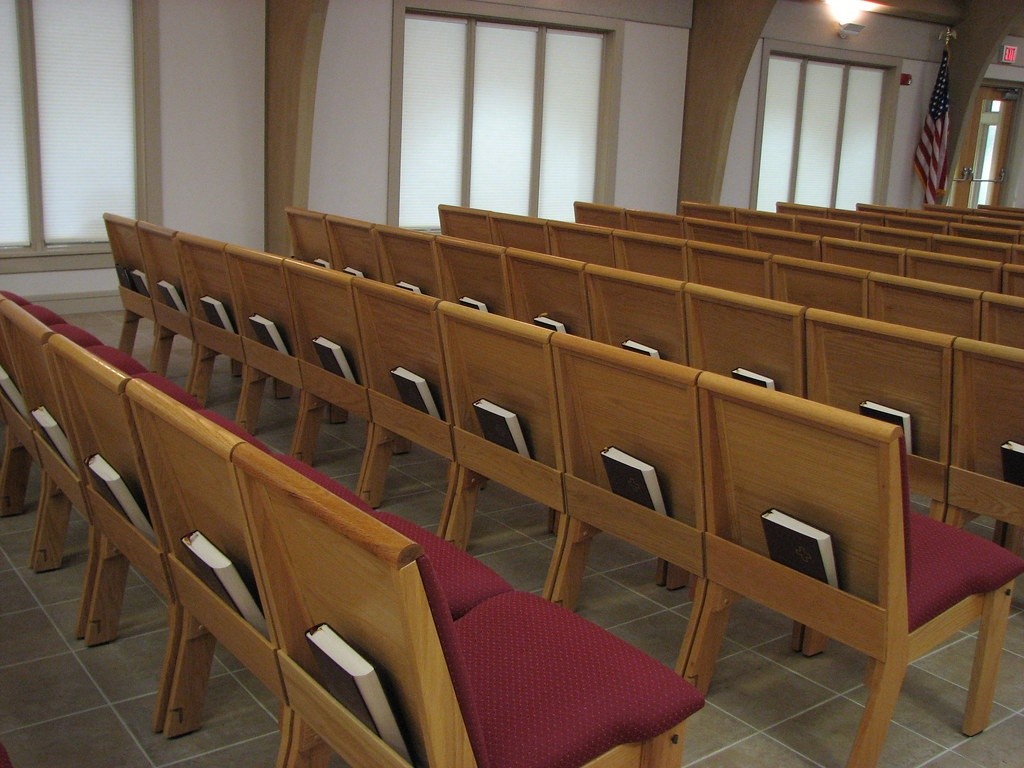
[913, 48, 950, 203]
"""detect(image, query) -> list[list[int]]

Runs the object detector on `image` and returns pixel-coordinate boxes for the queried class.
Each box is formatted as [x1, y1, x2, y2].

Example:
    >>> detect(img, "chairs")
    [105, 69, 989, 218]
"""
[0, 199, 1024, 768]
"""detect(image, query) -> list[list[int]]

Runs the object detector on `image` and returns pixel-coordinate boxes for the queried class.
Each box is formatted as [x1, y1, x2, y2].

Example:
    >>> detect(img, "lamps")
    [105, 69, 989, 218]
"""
[839, 22, 865, 40]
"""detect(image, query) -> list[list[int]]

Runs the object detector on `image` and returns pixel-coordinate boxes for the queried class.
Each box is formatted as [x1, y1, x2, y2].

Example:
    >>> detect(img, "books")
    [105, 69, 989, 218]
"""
[0, 259, 1024, 768]
